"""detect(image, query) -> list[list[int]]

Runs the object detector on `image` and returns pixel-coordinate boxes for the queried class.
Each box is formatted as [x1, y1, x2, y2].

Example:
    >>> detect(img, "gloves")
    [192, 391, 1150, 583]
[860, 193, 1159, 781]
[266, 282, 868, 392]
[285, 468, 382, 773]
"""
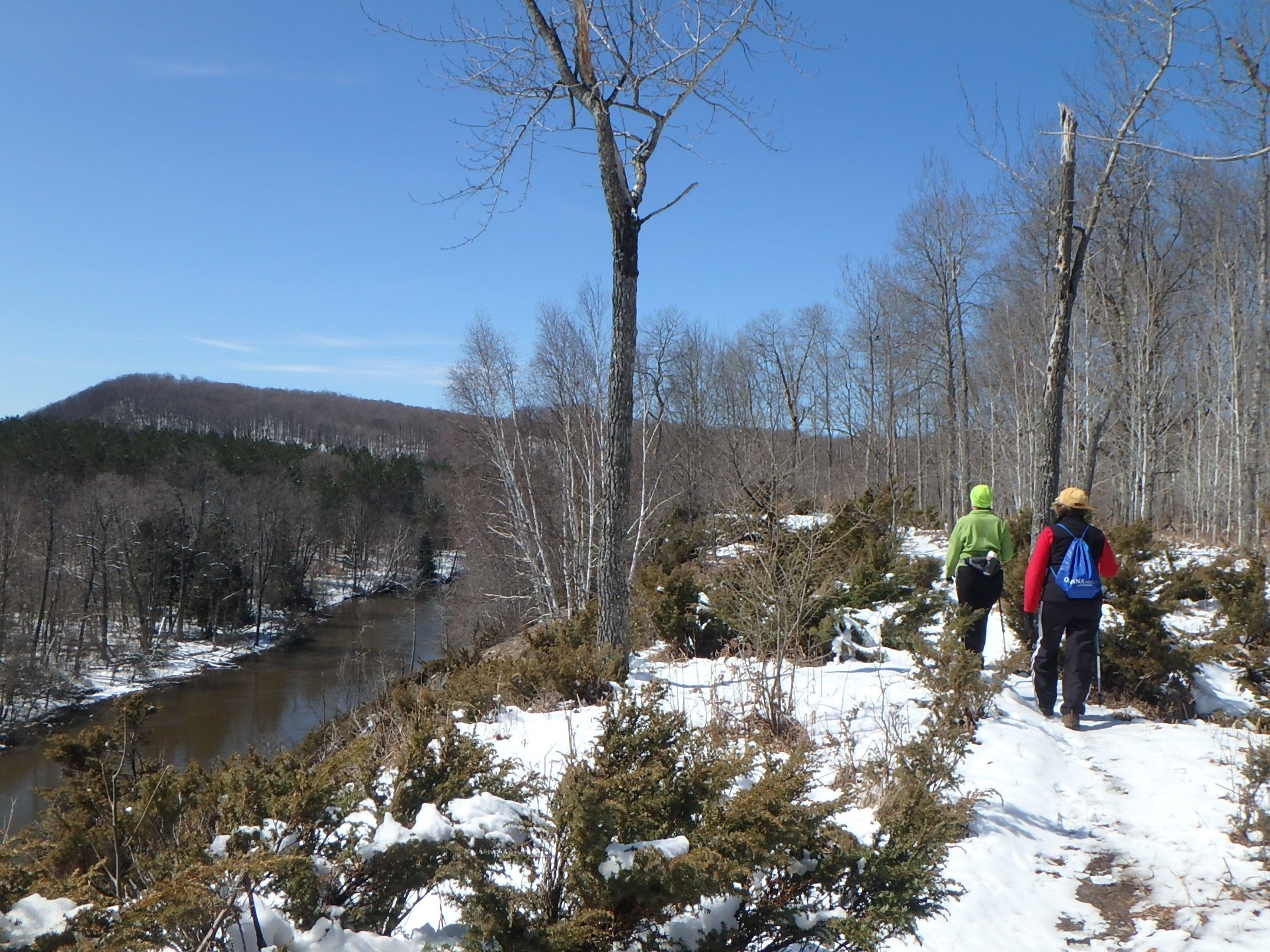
[1023, 612, 1037, 631]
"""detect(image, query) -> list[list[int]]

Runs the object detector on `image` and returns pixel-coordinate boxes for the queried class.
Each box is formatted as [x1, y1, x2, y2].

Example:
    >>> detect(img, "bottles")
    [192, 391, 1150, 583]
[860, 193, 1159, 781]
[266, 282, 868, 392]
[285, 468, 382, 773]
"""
[983, 549, 998, 576]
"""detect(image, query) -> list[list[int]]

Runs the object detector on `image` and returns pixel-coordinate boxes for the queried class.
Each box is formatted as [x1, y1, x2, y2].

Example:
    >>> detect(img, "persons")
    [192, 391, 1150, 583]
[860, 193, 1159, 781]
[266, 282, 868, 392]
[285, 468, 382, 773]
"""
[1023, 486, 1117, 729]
[945, 485, 1014, 655]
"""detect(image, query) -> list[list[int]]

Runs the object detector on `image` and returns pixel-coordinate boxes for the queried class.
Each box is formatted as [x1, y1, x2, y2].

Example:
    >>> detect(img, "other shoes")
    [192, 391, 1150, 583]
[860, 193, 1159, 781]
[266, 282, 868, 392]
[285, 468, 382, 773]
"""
[1062, 712, 1079, 729]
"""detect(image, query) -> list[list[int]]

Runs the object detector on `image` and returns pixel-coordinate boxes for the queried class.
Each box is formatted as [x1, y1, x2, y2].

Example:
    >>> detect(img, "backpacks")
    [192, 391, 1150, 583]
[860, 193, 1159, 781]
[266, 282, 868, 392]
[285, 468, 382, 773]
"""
[1049, 523, 1101, 599]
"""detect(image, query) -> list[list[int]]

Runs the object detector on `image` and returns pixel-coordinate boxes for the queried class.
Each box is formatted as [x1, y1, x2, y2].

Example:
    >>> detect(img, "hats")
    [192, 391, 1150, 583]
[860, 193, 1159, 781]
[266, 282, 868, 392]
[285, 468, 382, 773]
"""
[970, 483, 992, 507]
[1056, 487, 1095, 511]
